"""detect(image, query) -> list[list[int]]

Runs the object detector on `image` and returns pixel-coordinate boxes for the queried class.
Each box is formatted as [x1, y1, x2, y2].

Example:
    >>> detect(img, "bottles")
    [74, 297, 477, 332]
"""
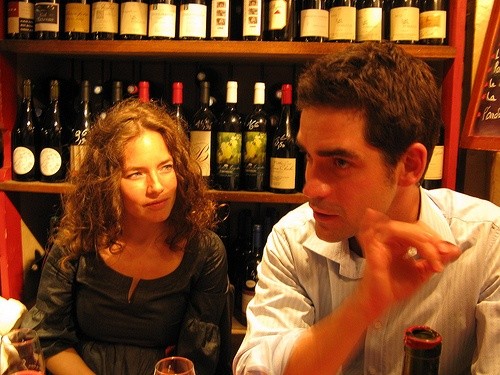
[402, 325, 442, 375]
[11, 78, 305, 195]
[6, 0, 446, 46]
[421, 83, 444, 190]
[216, 203, 289, 327]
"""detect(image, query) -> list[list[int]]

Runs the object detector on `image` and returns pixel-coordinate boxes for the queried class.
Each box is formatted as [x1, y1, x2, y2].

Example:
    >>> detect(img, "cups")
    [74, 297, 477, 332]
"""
[153, 356, 195, 375]
[2, 328, 46, 375]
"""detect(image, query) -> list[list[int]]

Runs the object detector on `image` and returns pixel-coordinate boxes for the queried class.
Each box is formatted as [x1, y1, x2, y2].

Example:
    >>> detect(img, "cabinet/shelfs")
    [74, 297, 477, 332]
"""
[0, 0, 466, 336]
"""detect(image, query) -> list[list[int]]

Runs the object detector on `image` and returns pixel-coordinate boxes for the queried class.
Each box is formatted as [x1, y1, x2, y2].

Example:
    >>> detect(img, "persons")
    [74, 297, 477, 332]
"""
[20, 96, 235, 375]
[233, 40, 500, 375]
